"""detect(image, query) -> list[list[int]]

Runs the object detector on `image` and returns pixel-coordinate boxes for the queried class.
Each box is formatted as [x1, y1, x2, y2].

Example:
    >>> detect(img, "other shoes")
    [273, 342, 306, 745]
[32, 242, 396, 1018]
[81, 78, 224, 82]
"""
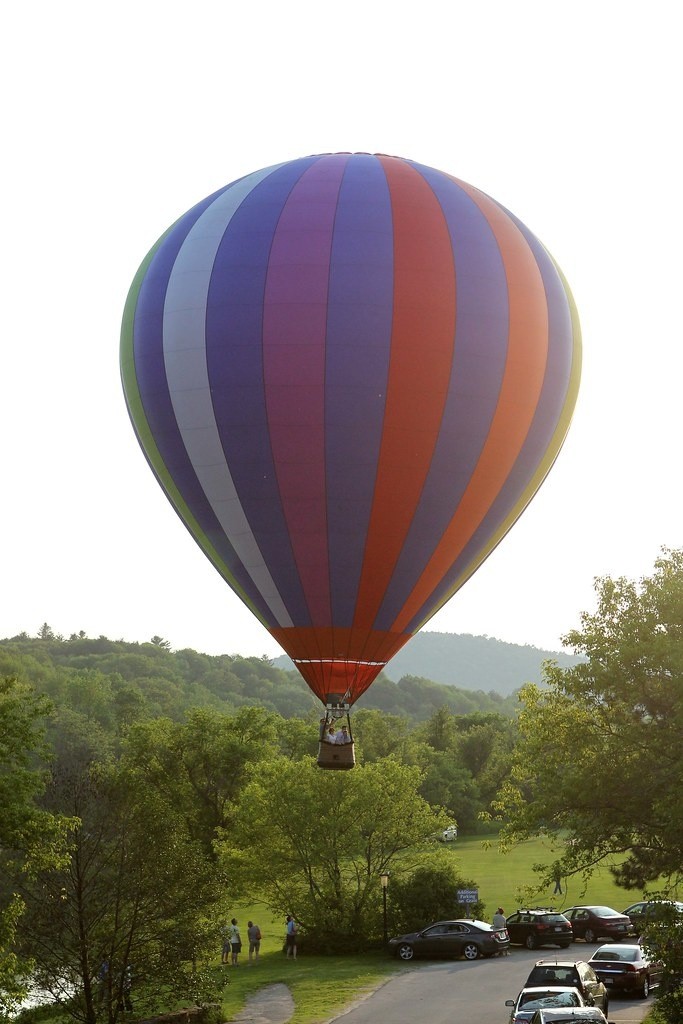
[233, 962, 240, 966]
[499, 952, 511, 955]
[286, 956, 297, 959]
[222, 961, 228, 964]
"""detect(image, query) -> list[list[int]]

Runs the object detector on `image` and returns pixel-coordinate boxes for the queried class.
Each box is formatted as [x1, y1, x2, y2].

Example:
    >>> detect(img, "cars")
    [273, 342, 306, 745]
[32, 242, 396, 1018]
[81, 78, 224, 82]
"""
[588, 945, 667, 999]
[562, 905, 633, 943]
[505, 987, 588, 1024]
[621, 901, 683, 932]
[531, 1006, 615, 1024]
[388, 918, 510, 961]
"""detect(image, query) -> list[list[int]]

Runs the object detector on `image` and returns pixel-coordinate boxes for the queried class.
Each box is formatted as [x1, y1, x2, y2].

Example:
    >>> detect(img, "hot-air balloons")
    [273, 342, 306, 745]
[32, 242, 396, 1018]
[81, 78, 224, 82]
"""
[115, 151, 584, 771]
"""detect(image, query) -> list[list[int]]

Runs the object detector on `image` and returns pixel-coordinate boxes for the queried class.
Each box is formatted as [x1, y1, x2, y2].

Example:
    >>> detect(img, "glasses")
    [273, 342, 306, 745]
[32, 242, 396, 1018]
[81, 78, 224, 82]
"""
[322, 721, 325, 722]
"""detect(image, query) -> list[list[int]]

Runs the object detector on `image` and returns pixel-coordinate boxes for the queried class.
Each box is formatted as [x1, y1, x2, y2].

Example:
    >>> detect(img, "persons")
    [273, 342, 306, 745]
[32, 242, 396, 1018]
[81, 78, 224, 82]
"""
[319, 717, 351, 744]
[553, 862, 564, 895]
[221, 917, 260, 967]
[285, 916, 302, 960]
[493, 907, 510, 956]
[96, 954, 133, 1013]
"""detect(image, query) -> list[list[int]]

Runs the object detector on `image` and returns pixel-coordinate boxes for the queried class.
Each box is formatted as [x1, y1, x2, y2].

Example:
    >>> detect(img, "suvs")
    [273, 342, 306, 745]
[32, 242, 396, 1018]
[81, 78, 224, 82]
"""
[505, 907, 574, 950]
[523, 959, 609, 1020]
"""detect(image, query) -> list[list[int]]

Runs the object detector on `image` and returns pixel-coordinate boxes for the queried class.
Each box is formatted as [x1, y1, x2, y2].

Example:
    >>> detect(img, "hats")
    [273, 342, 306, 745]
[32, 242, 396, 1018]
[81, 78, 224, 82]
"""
[498, 907, 503, 914]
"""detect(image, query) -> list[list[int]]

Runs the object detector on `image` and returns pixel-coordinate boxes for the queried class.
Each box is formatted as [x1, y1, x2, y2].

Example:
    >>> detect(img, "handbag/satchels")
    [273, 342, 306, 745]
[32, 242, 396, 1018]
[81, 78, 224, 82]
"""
[291, 931, 296, 935]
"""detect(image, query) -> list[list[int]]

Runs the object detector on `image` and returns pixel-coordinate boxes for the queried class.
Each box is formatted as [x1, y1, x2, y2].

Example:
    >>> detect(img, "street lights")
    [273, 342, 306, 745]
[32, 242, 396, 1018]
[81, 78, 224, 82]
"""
[379, 873, 389, 956]
